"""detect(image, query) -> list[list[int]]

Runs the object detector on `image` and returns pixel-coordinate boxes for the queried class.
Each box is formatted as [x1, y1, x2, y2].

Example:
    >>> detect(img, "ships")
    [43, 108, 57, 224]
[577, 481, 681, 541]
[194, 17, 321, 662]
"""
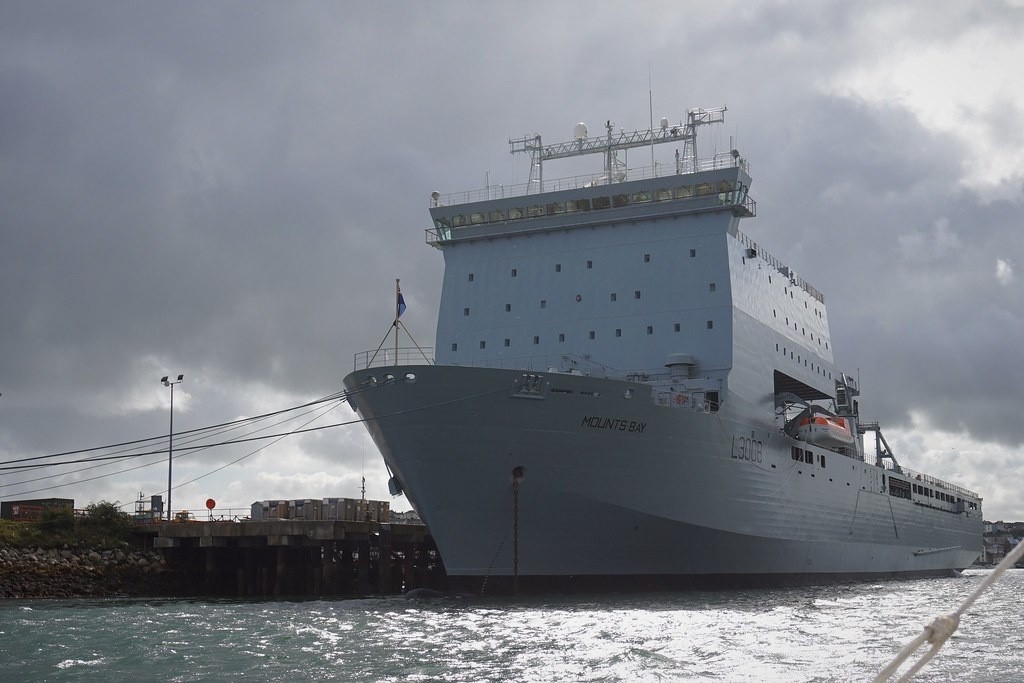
[340, 60, 983, 596]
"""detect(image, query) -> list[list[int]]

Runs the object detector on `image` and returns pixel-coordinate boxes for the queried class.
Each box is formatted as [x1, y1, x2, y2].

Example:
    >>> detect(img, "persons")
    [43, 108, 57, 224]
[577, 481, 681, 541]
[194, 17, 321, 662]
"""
[857, 455, 922, 481]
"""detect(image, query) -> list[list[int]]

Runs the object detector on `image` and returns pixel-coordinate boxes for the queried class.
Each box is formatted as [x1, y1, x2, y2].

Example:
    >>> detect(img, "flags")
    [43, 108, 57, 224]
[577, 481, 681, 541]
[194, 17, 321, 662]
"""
[392, 285, 407, 330]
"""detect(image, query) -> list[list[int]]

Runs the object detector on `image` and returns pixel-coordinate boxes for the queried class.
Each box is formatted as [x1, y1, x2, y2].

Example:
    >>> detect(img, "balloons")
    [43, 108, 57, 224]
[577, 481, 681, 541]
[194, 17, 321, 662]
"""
[205, 498, 215, 510]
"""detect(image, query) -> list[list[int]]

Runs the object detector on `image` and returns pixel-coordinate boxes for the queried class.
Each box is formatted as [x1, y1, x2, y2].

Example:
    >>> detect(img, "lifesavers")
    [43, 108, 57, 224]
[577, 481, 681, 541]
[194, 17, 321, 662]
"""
[676, 395, 685, 404]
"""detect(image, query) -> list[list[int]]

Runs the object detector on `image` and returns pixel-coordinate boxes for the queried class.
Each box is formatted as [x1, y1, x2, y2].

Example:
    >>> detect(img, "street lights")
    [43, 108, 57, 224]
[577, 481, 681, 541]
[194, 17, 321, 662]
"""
[160, 374, 185, 521]
